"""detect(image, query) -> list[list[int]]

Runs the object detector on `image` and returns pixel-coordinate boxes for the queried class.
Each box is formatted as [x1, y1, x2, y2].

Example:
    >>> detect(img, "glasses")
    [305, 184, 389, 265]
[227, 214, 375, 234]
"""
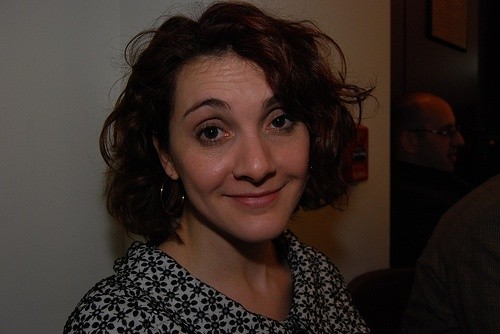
[414, 126, 459, 138]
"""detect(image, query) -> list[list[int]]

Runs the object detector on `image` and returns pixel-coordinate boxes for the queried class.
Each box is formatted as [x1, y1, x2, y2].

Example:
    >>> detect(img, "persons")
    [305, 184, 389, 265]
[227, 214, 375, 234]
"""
[388, 91, 468, 221]
[50, 1, 373, 334]
[414, 171, 500, 333]
